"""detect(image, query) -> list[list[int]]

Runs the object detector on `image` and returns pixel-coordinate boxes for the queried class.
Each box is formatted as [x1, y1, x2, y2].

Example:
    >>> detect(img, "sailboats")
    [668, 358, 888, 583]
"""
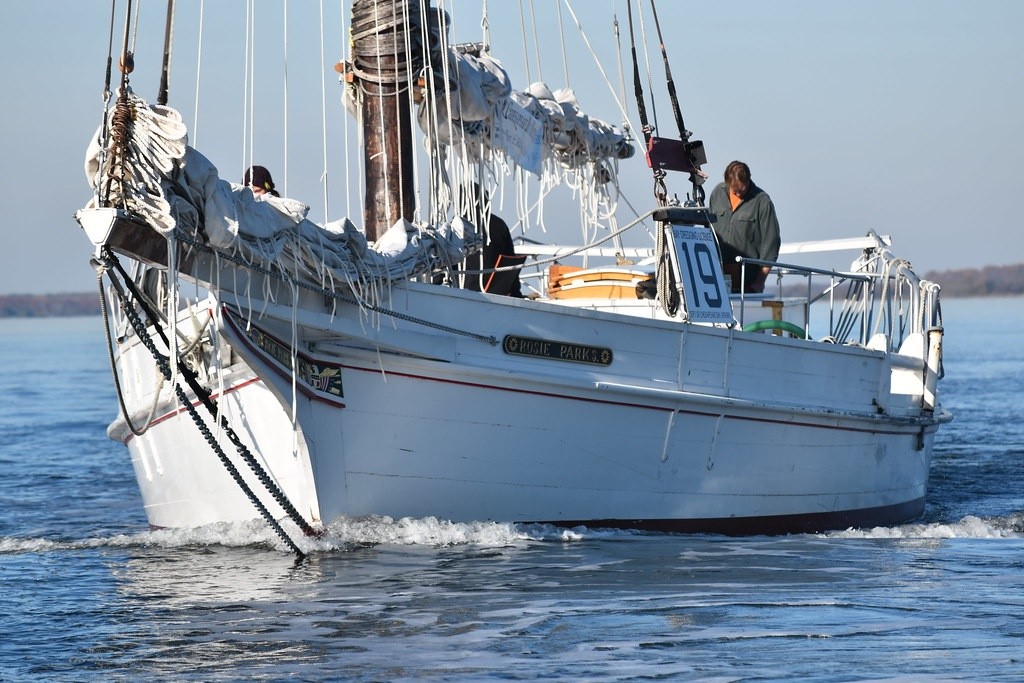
[73, 0, 946, 538]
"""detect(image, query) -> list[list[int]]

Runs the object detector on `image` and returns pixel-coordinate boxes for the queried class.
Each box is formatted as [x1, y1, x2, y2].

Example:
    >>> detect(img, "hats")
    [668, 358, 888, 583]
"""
[241, 165, 275, 191]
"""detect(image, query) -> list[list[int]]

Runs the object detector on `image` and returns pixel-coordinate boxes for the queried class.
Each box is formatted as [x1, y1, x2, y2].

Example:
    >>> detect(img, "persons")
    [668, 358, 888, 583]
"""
[709, 161, 781, 293]
[242, 167, 279, 197]
[434, 181, 522, 297]
